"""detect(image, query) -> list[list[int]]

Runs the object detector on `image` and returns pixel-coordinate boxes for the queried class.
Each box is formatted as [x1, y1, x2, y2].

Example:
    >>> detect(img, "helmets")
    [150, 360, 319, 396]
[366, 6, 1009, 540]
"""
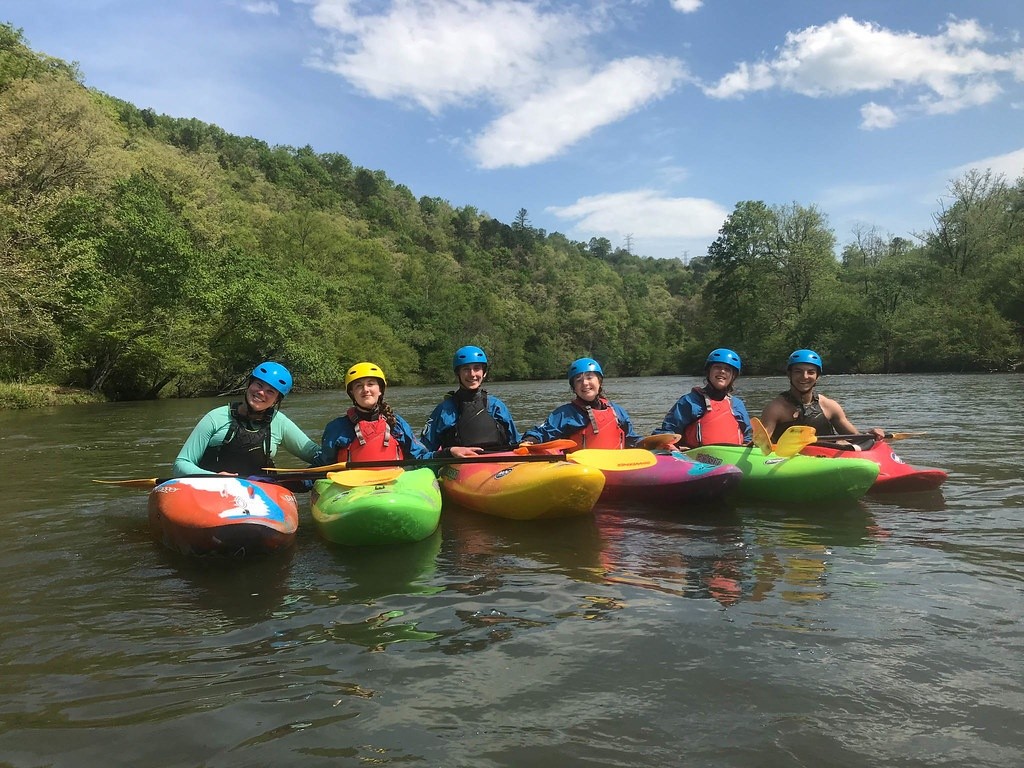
[345, 362, 386, 394]
[453, 346, 488, 372]
[568, 358, 604, 385]
[705, 348, 741, 375]
[787, 349, 822, 373]
[250, 362, 293, 396]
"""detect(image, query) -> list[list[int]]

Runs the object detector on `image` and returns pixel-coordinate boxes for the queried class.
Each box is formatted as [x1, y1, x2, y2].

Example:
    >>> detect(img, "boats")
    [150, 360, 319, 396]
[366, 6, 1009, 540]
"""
[771, 441, 947, 495]
[437, 451, 606, 522]
[563, 447, 743, 505]
[309, 466, 443, 547]
[149, 475, 300, 558]
[679, 444, 881, 503]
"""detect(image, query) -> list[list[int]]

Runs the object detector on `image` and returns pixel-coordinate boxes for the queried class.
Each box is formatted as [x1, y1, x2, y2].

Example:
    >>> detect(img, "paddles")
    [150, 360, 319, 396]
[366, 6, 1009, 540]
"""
[476, 438, 578, 454]
[637, 431, 682, 449]
[747, 414, 773, 457]
[90, 466, 406, 488]
[260, 447, 658, 473]
[774, 423, 927, 458]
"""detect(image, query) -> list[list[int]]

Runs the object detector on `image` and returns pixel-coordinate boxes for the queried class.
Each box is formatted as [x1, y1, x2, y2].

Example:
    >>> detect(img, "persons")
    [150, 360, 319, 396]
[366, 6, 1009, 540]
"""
[651, 348, 753, 450]
[172, 362, 324, 476]
[760, 349, 885, 442]
[278, 362, 485, 493]
[419, 345, 522, 453]
[520, 357, 678, 454]
